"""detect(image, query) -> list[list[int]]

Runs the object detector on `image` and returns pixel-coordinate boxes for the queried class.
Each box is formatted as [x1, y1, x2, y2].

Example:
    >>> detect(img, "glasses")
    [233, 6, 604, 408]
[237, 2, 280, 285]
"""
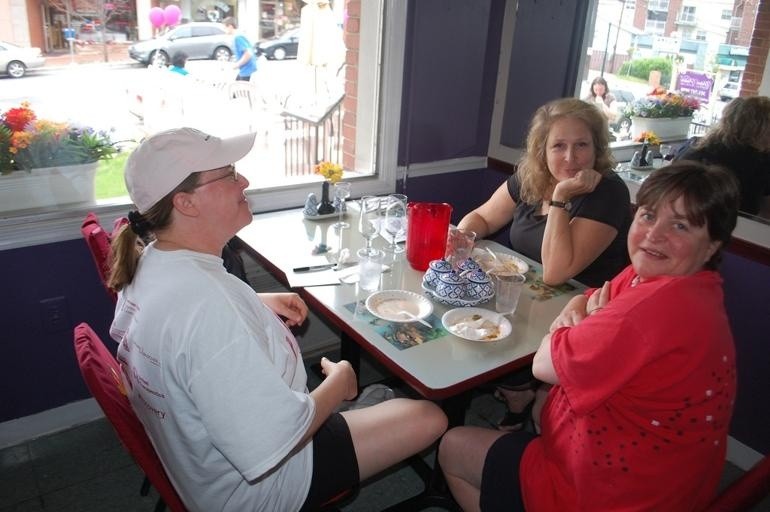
[191, 163, 239, 192]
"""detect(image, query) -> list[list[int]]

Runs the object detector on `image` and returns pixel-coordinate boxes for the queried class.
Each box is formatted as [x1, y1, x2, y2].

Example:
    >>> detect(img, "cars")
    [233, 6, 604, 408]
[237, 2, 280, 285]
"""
[606, 89, 636, 133]
[255, 25, 301, 61]
[127, 22, 236, 66]
[75, 23, 128, 46]
[0, 38, 46, 80]
[719, 81, 740, 103]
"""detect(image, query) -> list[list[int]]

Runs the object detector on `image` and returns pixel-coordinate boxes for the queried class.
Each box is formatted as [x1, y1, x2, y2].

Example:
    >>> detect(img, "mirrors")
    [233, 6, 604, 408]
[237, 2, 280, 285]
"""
[564, 0, 769, 226]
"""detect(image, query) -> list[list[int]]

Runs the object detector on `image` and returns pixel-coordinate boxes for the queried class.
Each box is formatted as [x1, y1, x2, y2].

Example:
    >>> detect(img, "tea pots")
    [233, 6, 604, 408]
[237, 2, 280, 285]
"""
[426, 254, 496, 300]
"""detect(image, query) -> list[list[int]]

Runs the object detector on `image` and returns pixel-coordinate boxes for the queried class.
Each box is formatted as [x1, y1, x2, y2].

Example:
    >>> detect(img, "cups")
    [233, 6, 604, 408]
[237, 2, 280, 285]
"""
[354, 248, 385, 292]
[404, 199, 453, 271]
[446, 228, 477, 262]
[494, 270, 525, 317]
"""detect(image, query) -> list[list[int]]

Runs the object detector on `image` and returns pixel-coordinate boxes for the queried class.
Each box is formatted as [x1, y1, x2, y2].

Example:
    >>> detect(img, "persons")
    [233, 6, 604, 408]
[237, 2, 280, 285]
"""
[584, 77, 617, 123]
[437, 160, 743, 511]
[671, 94, 770, 223]
[446, 97, 632, 434]
[171, 49, 190, 77]
[224, 18, 260, 99]
[108, 126, 449, 511]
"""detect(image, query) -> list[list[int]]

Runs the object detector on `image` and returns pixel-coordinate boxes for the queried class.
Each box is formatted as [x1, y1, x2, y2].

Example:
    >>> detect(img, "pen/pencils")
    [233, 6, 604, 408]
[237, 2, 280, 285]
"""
[293, 264, 337, 272]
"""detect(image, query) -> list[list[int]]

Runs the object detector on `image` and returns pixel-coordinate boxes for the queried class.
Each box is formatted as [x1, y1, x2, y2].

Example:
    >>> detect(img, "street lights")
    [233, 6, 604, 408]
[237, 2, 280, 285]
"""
[609, 0, 637, 73]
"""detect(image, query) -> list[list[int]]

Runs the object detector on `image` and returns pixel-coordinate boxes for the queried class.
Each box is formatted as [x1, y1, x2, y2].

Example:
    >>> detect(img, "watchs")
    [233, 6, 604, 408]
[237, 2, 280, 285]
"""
[548, 199, 573, 211]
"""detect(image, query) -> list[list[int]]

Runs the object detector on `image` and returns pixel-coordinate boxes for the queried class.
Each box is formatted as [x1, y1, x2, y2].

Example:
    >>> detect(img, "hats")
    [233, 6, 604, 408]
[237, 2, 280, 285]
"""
[122, 126, 257, 215]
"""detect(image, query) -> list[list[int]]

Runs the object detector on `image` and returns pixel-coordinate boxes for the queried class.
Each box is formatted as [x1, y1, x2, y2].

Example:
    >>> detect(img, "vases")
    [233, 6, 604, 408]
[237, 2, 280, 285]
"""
[317, 181, 336, 215]
[639, 144, 651, 167]
[0, 156, 97, 216]
[628, 113, 692, 141]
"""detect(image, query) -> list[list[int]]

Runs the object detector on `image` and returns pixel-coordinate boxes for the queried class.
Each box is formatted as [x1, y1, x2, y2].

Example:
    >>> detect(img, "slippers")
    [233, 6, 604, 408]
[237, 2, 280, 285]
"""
[491, 392, 538, 437]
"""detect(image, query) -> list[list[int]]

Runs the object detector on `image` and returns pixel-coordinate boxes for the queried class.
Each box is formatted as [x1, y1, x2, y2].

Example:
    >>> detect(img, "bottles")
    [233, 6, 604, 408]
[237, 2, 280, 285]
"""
[645, 150, 653, 166]
[303, 192, 317, 215]
[631, 151, 641, 166]
[334, 194, 345, 212]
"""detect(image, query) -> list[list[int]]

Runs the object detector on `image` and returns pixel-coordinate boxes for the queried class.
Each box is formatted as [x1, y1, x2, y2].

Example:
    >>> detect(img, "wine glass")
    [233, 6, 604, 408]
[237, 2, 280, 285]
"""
[334, 181, 351, 229]
[358, 194, 387, 262]
[659, 143, 671, 167]
[383, 190, 409, 253]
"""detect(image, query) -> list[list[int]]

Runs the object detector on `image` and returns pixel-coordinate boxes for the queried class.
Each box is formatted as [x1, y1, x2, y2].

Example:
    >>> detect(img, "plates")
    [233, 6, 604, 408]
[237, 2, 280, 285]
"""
[363, 289, 435, 324]
[418, 272, 496, 306]
[442, 306, 512, 344]
[475, 250, 532, 279]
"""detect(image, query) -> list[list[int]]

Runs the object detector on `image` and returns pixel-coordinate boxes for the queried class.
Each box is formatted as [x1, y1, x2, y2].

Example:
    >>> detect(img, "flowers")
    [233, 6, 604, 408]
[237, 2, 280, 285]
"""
[312, 160, 343, 184]
[0, 103, 123, 170]
[625, 83, 702, 121]
[632, 129, 659, 146]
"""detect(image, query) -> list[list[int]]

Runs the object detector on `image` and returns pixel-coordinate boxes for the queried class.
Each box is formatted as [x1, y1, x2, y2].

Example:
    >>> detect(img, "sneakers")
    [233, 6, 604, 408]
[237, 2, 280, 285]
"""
[348, 382, 396, 414]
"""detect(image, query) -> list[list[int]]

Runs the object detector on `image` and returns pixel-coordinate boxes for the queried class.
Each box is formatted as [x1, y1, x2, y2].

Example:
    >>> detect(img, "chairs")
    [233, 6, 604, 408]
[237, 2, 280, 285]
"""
[81, 208, 125, 312]
[712, 453, 770, 510]
[71, 321, 359, 512]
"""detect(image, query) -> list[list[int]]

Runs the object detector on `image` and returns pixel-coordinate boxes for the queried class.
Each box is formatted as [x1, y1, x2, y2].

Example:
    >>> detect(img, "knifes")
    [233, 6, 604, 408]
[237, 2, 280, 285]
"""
[293, 261, 358, 275]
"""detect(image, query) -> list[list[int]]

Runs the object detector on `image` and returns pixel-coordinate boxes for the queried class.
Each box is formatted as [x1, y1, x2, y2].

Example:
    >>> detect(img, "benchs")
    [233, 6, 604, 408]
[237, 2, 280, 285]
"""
[454, 173, 768, 453]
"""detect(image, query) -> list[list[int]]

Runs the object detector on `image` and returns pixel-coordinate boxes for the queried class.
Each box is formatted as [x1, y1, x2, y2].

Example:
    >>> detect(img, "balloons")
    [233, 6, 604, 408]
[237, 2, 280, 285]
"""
[146, 7, 166, 28]
[165, 4, 182, 28]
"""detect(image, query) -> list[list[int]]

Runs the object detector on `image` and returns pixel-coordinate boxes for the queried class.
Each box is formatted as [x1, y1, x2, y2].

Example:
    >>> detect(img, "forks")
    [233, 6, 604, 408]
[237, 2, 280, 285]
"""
[386, 305, 432, 330]
